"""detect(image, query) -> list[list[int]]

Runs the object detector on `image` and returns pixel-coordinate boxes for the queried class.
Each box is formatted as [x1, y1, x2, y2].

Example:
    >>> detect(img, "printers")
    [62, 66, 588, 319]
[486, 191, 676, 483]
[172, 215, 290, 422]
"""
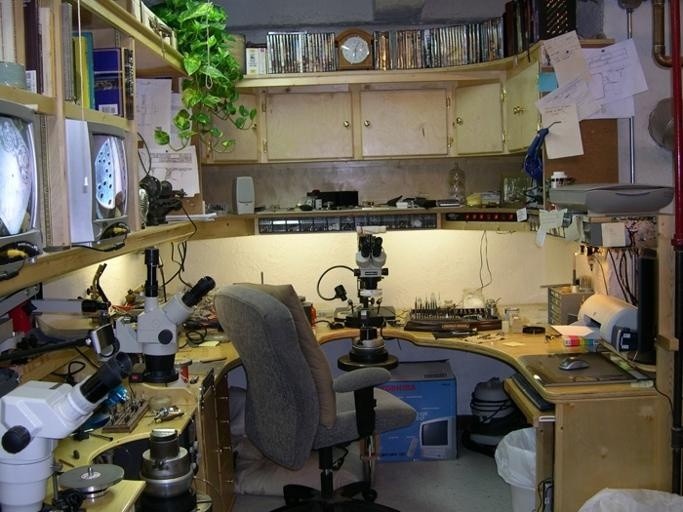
[550, 293, 638, 344]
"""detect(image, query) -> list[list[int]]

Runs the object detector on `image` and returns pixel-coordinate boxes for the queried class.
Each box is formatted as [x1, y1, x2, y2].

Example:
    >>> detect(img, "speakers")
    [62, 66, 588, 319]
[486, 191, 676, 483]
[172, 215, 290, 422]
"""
[233, 176, 255, 214]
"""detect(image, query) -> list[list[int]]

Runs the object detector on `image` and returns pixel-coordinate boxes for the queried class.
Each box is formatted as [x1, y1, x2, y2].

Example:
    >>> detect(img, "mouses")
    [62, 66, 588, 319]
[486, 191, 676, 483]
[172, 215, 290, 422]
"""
[558, 356, 592, 372]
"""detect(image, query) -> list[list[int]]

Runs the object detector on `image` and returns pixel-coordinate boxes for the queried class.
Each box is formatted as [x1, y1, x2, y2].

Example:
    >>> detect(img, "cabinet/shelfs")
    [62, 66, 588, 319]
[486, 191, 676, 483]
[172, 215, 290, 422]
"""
[196, 67, 263, 167]
[0, 0, 69, 299]
[256, 66, 452, 166]
[132, 20, 197, 254]
[62, 0, 144, 273]
[503, 35, 620, 157]
[449, 60, 508, 161]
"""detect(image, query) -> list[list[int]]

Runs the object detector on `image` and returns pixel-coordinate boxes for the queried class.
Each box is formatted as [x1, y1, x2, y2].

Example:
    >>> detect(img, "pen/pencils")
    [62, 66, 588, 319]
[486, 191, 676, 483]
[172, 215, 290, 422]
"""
[59, 459, 74, 468]
[200, 357, 228, 363]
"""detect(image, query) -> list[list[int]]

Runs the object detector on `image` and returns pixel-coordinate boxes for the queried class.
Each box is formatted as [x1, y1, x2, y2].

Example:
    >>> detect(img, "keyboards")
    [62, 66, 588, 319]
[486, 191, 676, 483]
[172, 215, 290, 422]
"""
[511, 373, 555, 412]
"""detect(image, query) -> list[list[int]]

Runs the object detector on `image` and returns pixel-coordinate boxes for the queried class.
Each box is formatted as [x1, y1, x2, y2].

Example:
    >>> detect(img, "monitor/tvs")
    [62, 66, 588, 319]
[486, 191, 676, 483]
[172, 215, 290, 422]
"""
[419, 416, 457, 460]
[66, 119, 131, 249]
[0, 100, 48, 274]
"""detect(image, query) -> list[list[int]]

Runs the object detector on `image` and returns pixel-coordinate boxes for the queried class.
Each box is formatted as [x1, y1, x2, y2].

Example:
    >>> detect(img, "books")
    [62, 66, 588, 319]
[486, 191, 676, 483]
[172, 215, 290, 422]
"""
[0, 1, 134, 128]
[263, 0, 580, 80]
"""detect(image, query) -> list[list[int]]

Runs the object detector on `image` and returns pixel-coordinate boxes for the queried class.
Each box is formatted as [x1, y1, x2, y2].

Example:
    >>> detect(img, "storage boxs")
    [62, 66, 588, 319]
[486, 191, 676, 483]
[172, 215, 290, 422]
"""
[368, 356, 462, 465]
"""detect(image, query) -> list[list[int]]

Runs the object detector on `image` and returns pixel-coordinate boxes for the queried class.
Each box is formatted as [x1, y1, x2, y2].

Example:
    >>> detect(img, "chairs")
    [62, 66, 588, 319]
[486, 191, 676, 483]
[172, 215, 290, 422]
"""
[212, 282, 423, 511]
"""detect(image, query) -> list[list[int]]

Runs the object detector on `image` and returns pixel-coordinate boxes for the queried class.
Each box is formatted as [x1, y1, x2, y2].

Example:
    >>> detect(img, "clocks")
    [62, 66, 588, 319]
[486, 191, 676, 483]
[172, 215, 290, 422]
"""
[334, 25, 377, 70]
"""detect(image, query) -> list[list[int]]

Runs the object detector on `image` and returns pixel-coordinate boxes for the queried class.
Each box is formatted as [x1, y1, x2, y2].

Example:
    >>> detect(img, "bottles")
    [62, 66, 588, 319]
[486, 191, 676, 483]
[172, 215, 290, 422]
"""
[500, 306, 523, 334]
[550, 171, 567, 191]
[449, 160, 466, 206]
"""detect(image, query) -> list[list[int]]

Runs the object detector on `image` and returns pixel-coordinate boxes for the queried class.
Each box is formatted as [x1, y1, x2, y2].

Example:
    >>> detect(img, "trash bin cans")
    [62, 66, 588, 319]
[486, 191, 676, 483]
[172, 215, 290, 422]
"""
[503, 427, 537, 512]
[577, 487, 683, 512]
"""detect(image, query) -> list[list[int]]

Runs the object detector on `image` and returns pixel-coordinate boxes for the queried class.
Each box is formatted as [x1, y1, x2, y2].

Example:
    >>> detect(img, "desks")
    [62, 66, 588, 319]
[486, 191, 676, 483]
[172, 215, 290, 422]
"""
[42, 300, 676, 512]
